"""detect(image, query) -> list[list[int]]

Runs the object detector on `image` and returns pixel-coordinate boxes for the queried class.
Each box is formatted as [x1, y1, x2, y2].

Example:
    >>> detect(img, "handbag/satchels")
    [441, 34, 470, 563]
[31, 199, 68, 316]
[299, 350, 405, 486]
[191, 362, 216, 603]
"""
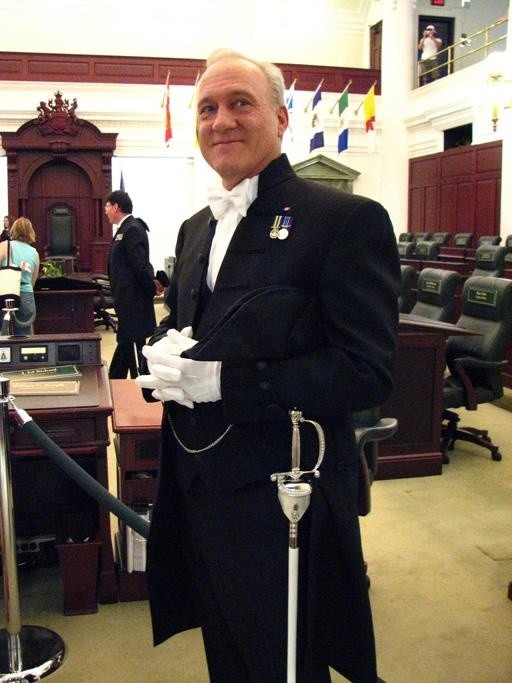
[0, 266, 22, 300]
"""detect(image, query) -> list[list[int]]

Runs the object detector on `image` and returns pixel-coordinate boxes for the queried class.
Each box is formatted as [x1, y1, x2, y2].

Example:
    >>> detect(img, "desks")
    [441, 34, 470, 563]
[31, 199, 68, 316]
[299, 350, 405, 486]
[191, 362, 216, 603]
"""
[3, 330, 102, 367]
[375, 313, 484, 482]
[3, 360, 119, 604]
[108, 378, 164, 602]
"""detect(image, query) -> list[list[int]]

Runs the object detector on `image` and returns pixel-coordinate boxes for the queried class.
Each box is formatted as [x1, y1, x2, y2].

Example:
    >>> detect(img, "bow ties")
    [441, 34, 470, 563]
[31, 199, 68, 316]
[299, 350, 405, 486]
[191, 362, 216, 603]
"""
[207, 178, 250, 220]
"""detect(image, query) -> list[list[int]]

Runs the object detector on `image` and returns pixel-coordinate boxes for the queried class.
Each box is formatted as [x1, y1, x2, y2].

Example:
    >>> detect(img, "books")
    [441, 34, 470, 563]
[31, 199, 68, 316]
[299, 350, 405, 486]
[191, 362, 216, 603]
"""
[1, 364, 82, 397]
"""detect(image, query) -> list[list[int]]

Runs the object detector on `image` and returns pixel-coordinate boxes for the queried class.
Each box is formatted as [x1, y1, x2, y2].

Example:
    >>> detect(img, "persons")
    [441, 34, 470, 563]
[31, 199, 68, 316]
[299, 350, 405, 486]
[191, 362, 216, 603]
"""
[418, 24, 442, 85]
[135, 49, 402, 683]
[0, 217, 40, 335]
[105, 191, 165, 379]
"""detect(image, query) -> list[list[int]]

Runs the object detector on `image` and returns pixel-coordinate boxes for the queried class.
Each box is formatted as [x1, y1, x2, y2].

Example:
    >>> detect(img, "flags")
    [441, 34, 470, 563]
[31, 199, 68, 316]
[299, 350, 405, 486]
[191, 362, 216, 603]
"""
[363, 87, 376, 134]
[337, 90, 349, 155]
[309, 87, 325, 152]
[288, 84, 295, 139]
[194, 81, 200, 150]
[165, 76, 172, 142]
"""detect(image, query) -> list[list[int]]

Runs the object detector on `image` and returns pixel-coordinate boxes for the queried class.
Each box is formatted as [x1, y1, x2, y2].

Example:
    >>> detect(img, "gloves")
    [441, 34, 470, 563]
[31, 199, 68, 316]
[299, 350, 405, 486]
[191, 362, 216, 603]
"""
[134, 326, 223, 410]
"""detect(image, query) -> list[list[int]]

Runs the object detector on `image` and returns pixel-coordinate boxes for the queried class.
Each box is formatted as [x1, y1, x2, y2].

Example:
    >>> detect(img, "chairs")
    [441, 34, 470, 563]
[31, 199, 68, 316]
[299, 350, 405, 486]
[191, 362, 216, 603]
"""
[396, 231, 512, 466]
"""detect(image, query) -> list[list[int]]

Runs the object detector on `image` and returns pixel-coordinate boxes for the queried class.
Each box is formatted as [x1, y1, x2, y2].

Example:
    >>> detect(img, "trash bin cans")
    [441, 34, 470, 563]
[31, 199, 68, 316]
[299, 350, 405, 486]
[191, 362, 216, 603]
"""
[164, 256, 176, 280]
[53, 529, 101, 616]
